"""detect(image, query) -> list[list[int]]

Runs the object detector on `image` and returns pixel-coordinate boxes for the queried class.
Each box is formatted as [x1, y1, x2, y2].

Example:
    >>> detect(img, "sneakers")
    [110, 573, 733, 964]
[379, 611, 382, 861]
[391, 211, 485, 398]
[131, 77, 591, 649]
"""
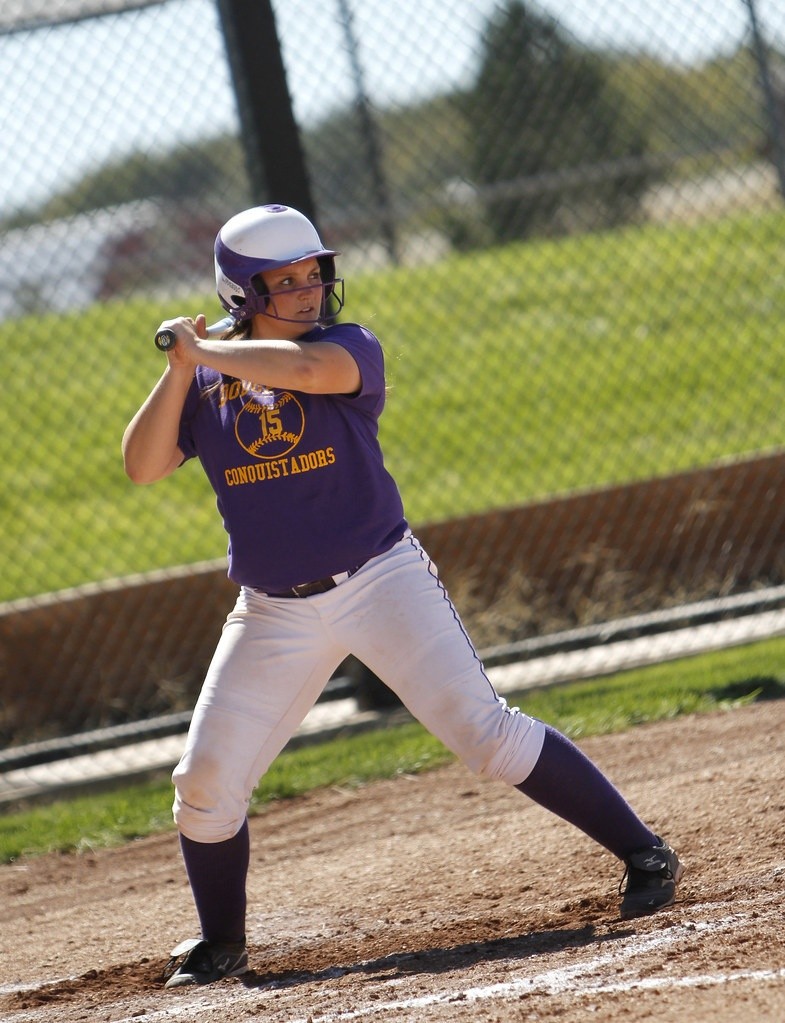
[619, 835, 686, 916]
[160, 938, 248, 988]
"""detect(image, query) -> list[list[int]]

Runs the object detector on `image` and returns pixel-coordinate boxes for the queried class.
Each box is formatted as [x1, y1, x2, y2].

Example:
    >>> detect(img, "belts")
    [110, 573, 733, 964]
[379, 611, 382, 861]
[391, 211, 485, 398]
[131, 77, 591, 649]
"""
[267, 563, 365, 598]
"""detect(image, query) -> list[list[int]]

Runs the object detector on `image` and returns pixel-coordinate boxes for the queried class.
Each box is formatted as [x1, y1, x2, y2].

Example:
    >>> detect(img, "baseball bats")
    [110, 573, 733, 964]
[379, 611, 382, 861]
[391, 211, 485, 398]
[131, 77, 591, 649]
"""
[153, 314, 236, 351]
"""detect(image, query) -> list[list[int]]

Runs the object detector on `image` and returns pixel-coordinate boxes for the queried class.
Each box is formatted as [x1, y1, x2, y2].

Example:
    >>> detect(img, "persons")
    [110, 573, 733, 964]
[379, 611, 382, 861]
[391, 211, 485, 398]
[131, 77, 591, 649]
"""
[120, 204, 684, 990]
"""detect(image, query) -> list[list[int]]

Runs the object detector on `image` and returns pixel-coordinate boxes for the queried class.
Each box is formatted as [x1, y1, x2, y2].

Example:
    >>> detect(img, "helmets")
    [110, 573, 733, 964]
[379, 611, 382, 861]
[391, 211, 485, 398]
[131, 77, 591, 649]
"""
[215, 203, 339, 317]
